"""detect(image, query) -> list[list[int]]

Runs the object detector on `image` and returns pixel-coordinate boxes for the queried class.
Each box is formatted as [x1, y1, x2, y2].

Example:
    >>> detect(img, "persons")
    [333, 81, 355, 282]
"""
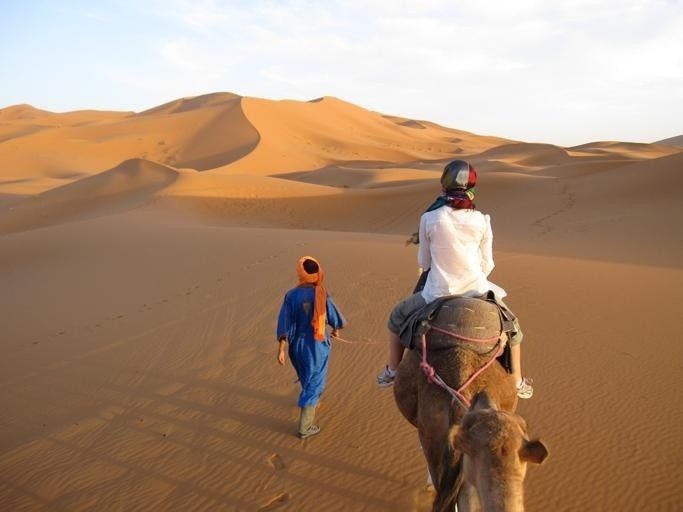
[276, 256, 345, 437]
[374, 160, 536, 399]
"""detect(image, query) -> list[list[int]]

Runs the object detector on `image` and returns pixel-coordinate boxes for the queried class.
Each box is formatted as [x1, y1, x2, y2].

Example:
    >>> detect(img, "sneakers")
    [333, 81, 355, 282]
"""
[299, 425, 320, 439]
[376, 365, 397, 385]
[515, 377, 534, 399]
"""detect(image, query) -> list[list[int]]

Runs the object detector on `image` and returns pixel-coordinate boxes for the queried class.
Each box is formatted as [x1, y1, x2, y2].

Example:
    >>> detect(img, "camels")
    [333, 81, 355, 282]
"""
[391, 229, 520, 512]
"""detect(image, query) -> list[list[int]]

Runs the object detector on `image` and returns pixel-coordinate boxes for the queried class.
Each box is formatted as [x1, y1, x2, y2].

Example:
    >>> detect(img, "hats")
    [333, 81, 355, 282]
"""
[441, 159, 479, 191]
